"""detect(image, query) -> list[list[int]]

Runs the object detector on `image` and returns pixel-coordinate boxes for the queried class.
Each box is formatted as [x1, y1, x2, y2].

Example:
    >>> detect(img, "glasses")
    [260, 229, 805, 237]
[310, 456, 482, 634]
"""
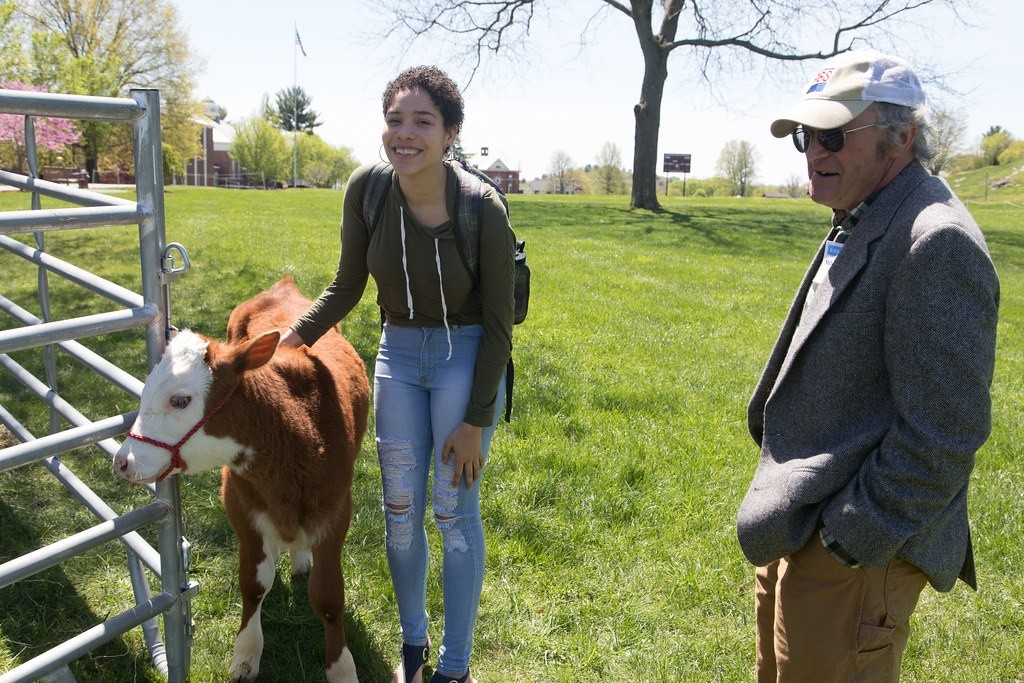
[790, 121, 878, 153]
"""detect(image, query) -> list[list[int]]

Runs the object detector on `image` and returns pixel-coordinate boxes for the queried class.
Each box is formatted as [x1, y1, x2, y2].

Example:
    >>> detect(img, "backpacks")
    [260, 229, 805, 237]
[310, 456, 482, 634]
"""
[362, 156, 529, 325]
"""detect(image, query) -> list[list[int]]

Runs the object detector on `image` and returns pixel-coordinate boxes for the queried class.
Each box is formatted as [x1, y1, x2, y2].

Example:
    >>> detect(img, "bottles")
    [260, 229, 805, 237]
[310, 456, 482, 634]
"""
[513, 240, 531, 325]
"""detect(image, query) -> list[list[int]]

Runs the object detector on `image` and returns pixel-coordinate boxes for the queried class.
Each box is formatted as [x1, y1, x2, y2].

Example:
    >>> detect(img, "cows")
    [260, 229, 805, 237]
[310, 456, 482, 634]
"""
[114, 275, 371, 683]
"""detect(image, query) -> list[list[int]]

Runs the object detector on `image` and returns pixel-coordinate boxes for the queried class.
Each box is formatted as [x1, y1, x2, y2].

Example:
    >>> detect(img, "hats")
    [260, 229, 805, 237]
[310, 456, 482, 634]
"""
[770, 51, 927, 139]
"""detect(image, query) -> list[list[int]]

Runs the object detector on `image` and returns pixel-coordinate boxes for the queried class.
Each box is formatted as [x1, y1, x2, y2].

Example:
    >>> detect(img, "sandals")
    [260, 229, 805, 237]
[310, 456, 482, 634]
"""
[389, 631, 432, 683]
[430, 667, 469, 683]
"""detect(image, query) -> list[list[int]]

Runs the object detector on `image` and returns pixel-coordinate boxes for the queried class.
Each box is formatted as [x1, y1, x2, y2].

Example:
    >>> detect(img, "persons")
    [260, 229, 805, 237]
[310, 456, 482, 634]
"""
[278, 66, 516, 683]
[737, 50, 1000, 683]
